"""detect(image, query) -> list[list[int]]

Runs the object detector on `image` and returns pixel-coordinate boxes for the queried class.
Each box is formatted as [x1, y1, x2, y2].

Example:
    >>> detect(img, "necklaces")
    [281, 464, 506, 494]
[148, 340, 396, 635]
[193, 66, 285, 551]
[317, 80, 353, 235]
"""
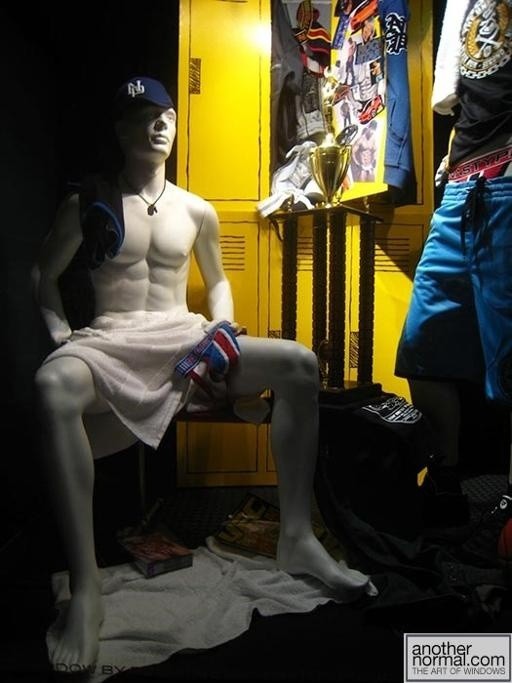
[120, 172, 166, 215]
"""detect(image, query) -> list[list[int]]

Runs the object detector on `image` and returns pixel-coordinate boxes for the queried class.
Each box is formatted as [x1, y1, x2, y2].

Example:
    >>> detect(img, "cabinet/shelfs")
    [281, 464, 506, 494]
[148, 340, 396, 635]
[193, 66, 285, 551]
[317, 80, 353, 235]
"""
[176, 0, 434, 487]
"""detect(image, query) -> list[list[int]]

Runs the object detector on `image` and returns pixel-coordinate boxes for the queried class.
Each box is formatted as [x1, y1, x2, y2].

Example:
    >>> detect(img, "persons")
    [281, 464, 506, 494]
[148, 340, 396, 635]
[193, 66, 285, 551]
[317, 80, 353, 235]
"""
[28, 77, 371, 675]
[394, 0, 511, 561]
[333, 20, 385, 183]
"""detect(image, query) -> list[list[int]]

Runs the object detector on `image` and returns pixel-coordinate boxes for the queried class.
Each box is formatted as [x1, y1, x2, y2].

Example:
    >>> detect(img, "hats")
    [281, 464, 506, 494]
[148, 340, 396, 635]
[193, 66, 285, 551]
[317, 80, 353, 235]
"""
[113, 77, 176, 122]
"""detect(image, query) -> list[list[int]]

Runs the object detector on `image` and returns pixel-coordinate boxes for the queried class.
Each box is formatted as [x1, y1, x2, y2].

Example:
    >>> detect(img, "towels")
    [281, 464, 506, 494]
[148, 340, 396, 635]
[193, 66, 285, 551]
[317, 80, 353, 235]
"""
[49, 528, 379, 683]
[41, 310, 231, 450]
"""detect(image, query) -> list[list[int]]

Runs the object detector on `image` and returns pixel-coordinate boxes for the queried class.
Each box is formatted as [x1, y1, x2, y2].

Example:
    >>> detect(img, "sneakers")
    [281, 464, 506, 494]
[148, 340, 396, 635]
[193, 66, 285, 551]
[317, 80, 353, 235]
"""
[461, 490, 512, 566]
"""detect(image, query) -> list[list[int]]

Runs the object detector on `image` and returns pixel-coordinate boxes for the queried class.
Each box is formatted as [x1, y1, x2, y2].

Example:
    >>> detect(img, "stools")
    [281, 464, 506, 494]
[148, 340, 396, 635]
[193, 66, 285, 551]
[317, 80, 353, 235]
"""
[110, 381, 390, 579]
